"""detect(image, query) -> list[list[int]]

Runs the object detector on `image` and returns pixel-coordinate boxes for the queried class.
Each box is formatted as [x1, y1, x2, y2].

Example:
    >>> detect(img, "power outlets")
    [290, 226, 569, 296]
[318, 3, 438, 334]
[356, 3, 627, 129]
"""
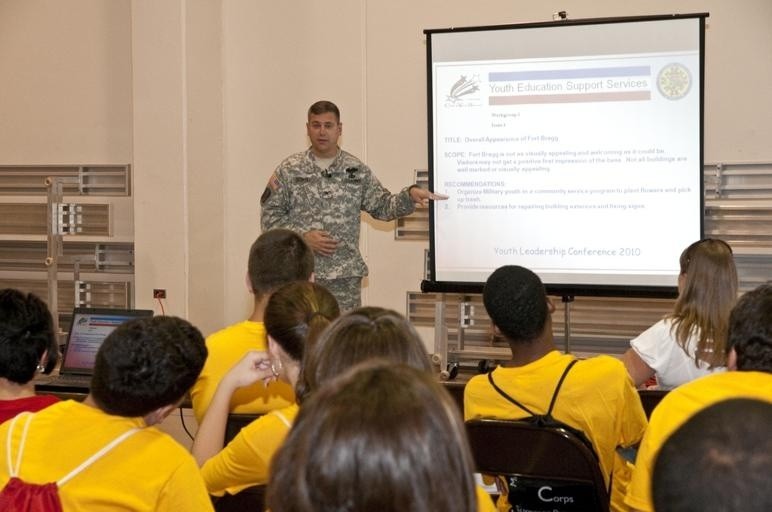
[154, 289, 165, 298]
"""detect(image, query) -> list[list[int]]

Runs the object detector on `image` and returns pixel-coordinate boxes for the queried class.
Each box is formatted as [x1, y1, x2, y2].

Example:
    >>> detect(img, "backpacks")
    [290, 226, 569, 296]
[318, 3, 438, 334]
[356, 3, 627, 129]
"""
[488, 360, 609, 511]
[1, 412, 147, 512]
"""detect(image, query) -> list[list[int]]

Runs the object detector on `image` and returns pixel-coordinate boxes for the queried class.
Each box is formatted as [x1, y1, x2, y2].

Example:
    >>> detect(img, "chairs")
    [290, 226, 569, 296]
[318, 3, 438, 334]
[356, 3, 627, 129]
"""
[629, 391, 671, 451]
[463, 419, 610, 512]
[210, 414, 270, 512]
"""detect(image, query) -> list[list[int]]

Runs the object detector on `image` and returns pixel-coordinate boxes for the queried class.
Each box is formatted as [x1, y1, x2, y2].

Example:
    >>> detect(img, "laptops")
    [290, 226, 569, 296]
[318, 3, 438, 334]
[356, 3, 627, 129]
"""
[34, 307, 154, 394]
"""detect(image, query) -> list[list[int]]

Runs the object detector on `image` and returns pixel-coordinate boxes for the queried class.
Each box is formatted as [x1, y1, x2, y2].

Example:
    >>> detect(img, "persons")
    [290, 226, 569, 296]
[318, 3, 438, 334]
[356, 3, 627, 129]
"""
[190, 225, 316, 428]
[0, 312, 218, 512]
[0, 284, 74, 416]
[190, 279, 347, 500]
[264, 361, 480, 511]
[619, 283, 771, 511]
[611, 236, 742, 392]
[648, 398, 772, 512]
[461, 260, 652, 512]
[291, 303, 437, 409]
[258, 98, 452, 321]
